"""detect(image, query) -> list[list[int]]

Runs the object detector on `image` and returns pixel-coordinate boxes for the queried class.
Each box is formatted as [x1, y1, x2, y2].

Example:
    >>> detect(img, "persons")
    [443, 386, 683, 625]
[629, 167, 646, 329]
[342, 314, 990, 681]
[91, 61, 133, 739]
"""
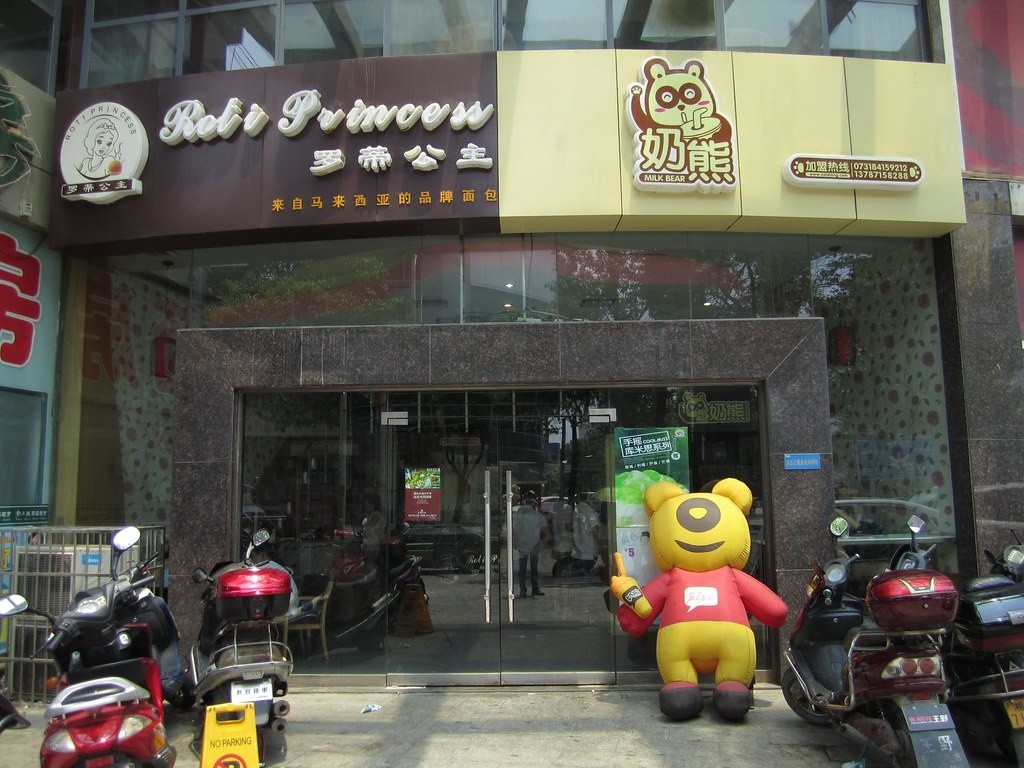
[358, 493, 387, 548]
[514, 483, 608, 600]
[517, 491, 548, 597]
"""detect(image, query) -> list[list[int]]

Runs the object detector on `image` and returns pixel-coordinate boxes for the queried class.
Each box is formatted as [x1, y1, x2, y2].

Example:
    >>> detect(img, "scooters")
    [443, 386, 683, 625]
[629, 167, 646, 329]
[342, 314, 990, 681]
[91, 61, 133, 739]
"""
[779, 514, 970, 768]
[0, 526, 196, 768]
[187, 528, 298, 768]
[270, 518, 426, 661]
[883, 513, 1024, 768]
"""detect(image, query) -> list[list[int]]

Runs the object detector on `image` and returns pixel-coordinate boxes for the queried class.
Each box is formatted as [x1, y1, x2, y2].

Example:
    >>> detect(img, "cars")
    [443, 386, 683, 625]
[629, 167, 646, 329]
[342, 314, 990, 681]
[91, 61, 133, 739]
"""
[833, 482, 1024, 589]
[405, 518, 499, 576]
[502, 494, 601, 544]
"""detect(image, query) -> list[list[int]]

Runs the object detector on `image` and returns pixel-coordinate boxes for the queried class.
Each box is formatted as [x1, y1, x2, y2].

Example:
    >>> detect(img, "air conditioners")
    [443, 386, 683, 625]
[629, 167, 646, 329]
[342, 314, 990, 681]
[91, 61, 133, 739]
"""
[8, 546, 139, 698]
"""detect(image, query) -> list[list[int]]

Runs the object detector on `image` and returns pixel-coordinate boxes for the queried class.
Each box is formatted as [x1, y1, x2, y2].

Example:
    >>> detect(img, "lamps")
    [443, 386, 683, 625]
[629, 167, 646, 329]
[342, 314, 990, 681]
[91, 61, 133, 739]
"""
[828, 246, 857, 366]
[150, 260, 177, 379]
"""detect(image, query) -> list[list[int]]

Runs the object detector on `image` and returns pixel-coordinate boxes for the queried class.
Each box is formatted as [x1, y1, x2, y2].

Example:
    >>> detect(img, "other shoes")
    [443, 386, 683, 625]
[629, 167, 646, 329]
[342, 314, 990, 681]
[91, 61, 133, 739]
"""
[532, 588, 544, 596]
[520, 592, 526, 598]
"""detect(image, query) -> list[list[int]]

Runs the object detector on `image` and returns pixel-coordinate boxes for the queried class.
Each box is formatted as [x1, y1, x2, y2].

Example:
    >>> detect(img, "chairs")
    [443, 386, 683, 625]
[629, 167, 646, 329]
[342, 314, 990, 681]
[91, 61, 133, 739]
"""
[282, 575, 334, 664]
